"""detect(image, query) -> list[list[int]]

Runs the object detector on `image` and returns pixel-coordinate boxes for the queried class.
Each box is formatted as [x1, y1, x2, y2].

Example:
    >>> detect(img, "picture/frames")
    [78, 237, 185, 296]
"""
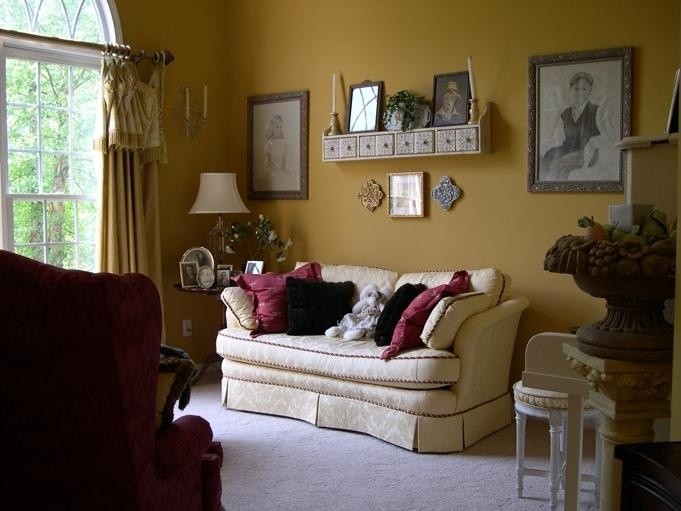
[387, 172, 424, 218]
[430, 71, 469, 128]
[179, 246, 232, 289]
[526, 46, 632, 193]
[247, 89, 308, 200]
[244, 261, 264, 274]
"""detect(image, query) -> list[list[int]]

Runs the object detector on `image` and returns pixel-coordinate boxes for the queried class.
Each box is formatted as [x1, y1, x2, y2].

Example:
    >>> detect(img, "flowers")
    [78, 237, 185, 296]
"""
[228, 214, 294, 262]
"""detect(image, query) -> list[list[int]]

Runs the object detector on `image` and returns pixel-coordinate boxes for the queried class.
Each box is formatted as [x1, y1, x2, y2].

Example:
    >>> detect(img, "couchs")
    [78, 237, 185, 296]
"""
[216, 261, 531, 452]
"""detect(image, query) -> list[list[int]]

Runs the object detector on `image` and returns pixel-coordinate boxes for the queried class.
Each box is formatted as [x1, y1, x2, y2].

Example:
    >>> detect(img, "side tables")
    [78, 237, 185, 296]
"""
[173, 283, 225, 386]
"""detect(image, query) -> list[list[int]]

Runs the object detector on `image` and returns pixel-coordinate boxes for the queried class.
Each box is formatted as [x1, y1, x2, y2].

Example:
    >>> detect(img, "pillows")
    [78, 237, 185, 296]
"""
[156, 344, 198, 434]
[231, 263, 469, 360]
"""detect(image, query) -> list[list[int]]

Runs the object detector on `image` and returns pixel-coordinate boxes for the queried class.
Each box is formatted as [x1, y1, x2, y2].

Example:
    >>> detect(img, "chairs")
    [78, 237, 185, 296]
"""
[0, 251, 221, 511]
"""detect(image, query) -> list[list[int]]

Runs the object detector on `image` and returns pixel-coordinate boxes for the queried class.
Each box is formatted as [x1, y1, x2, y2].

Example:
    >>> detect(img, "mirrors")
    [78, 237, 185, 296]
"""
[344, 79, 382, 134]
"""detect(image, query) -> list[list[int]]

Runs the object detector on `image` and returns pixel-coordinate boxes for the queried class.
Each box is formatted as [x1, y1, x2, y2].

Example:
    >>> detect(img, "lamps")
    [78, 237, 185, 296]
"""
[189, 173, 251, 265]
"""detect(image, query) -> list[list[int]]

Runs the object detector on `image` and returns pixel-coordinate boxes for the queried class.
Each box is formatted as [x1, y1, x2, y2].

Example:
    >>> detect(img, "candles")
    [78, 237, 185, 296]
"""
[333, 73, 335, 113]
[468, 56, 475, 98]
[204, 85, 207, 119]
[186, 88, 190, 121]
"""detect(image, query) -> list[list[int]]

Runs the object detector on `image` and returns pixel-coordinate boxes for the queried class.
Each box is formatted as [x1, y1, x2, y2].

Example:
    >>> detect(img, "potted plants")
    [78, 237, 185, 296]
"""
[380, 89, 432, 132]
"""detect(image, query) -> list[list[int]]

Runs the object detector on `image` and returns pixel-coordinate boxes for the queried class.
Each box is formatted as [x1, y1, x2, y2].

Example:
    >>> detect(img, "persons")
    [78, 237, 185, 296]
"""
[437, 82, 467, 121]
[259, 116, 294, 173]
[182, 263, 199, 286]
[219, 272, 228, 282]
[535, 70, 618, 181]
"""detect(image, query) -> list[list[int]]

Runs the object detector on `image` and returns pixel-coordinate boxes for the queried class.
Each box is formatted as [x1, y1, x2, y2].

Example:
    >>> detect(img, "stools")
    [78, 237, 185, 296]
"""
[513, 380, 600, 511]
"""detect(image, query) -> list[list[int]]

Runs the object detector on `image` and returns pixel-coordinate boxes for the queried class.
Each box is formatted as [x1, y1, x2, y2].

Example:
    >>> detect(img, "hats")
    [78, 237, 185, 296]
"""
[439, 81, 462, 105]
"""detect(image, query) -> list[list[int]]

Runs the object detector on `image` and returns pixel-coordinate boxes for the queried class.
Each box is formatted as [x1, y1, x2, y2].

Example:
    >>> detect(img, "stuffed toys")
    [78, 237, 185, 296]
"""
[324, 283, 392, 344]
